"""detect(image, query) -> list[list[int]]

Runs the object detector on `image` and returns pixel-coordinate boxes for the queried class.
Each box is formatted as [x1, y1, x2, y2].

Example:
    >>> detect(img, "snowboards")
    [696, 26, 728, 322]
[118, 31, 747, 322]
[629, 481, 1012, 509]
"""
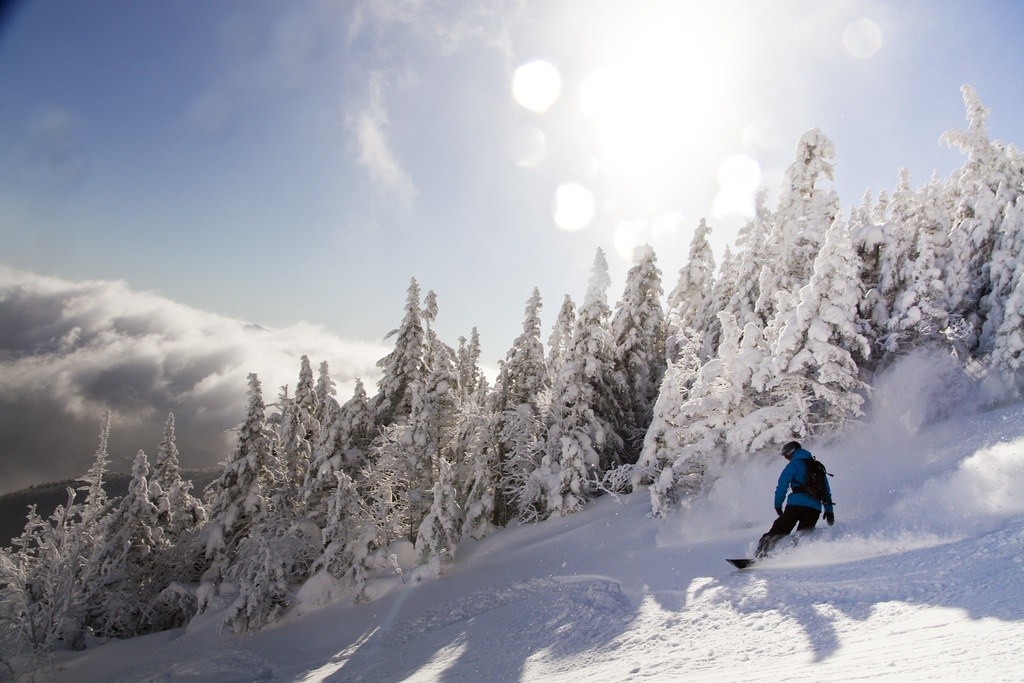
[725, 558, 754, 569]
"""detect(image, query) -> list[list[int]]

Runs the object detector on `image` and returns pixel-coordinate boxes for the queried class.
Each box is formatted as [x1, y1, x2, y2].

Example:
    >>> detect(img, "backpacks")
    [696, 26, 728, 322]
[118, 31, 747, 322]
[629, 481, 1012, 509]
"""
[789, 459, 828, 502]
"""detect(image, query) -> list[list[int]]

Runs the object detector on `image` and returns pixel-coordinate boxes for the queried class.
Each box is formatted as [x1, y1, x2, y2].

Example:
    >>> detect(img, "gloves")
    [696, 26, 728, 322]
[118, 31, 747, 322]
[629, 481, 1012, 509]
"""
[823, 511, 834, 526]
[775, 507, 783, 516]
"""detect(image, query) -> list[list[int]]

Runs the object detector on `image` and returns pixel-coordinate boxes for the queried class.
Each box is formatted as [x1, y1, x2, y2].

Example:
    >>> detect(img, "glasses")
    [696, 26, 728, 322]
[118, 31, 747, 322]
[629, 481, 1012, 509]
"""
[783, 451, 794, 458]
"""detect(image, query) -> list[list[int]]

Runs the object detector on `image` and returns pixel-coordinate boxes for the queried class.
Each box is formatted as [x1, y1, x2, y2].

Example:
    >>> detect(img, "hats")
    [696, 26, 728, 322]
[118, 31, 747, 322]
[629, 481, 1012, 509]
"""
[781, 440, 800, 455]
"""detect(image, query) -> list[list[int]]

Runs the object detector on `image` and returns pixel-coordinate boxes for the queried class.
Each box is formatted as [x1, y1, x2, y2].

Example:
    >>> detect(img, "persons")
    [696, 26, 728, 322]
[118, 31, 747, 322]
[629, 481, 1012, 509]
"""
[752, 441, 838, 561]
